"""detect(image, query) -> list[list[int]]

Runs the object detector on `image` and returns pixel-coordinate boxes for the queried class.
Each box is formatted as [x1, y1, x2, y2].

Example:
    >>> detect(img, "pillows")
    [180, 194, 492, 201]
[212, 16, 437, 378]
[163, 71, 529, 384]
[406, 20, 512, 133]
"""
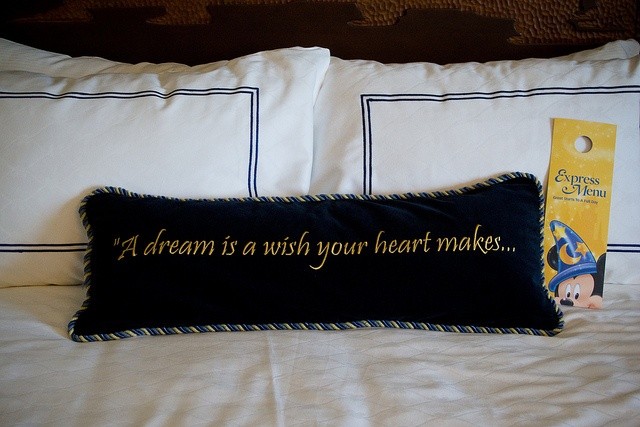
[1, 34, 333, 290]
[66, 172, 567, 344]
[313, 39, 640, 287]
